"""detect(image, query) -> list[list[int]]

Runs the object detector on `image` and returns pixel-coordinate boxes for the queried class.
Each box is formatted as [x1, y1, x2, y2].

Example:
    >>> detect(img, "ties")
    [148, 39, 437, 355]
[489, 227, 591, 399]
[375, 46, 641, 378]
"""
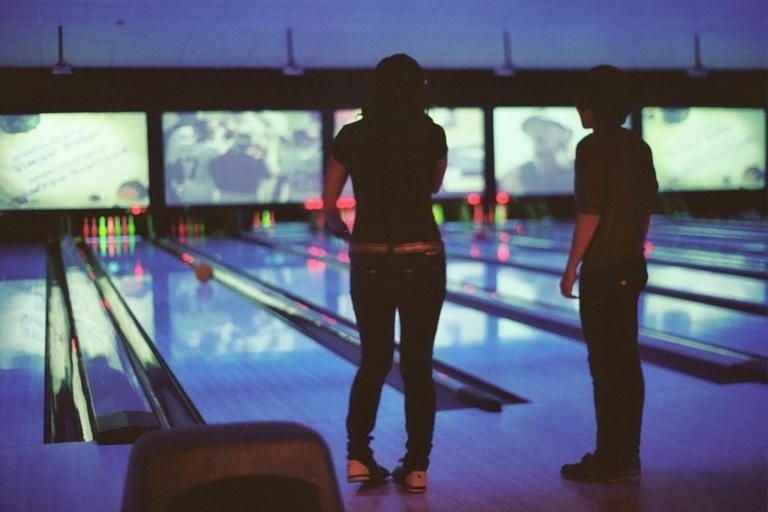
[353, 241, 442, 255]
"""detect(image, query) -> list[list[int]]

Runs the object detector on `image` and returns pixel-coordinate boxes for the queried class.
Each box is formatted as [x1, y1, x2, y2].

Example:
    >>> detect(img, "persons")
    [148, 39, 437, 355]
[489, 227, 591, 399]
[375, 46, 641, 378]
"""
[561, 66, 659, 482]
[322, 55, 447, 493]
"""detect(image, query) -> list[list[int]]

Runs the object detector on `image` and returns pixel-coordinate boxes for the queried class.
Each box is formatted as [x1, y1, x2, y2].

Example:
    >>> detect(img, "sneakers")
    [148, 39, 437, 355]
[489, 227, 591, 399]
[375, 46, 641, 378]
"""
[347, 457, 389, 483]
[561, 448, 639, 481]
[392, 465, 428, 492]
